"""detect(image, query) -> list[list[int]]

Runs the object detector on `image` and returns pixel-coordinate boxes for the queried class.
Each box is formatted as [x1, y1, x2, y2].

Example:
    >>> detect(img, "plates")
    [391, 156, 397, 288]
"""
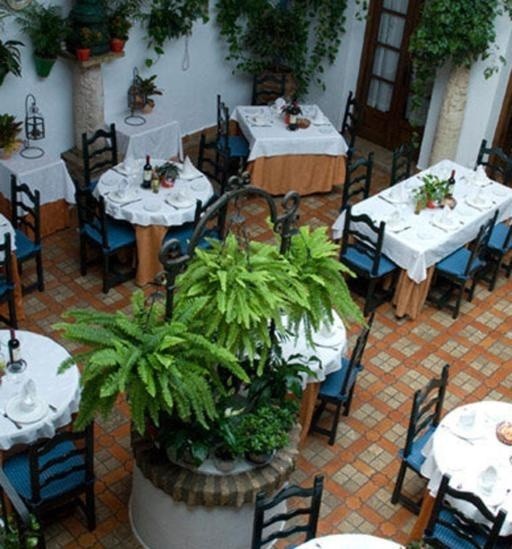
[380, 161, 496, 233]
[102, 158, 208, 210]
[5, 393, 48, 422]
[311, 322, 345, 347]
[242, 103, 331, 133]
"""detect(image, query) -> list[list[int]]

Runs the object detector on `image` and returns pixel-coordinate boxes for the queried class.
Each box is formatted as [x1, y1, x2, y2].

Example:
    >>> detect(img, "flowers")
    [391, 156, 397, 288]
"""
[110, 16, 131, 39]
[74, 28, 102, 49]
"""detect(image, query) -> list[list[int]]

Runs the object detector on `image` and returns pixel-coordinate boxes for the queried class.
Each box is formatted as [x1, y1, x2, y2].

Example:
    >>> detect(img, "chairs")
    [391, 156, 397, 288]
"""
[9, 175, 45, 296]
[164, 193, 230, 260]
[81, 122, 118, 184]
[390, 363, 450, 518]
[311, 312, 374, 446]
[212, 94, 250, 182]
[337, 92, 512, 319]
[252, 475, 324, 548]
[75, 179, 136, 292]
[0, 419, 96, 547]
[424, 474, 507, 549]
[197, 133, 228, 195]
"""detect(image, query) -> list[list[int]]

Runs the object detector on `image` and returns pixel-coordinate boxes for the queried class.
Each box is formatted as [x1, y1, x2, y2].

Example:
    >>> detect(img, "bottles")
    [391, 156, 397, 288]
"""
[447, 167, 456, 196]
[6, 327, 23, 369]
[289, 101, 299, 130]
[141, 154, 153, 186]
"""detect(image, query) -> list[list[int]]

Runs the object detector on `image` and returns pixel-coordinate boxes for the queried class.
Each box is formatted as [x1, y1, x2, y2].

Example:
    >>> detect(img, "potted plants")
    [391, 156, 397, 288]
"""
[17, 3, 68, 80]
[132, 74, 163, 113]
[213, 0, 372, 106]
[0, 113, 23, 160]
[52, 221, 369, 548]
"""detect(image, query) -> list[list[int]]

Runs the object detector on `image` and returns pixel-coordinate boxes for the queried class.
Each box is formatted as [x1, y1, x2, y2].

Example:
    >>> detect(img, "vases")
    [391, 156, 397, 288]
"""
[75, 47, 91, 61]
[110, 38, 124, 52]
